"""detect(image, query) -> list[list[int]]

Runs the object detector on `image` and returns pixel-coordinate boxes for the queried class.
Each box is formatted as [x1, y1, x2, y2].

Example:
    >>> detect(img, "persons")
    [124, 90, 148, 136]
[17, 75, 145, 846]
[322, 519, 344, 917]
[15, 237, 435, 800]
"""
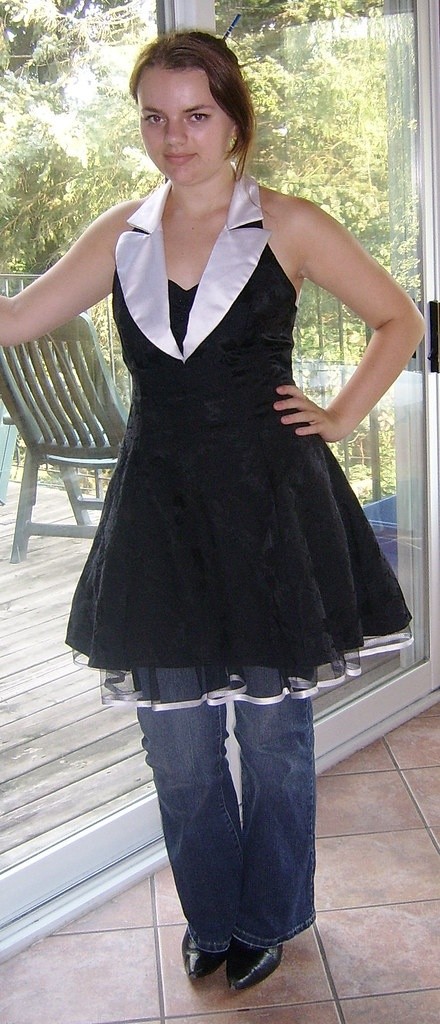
[0, 31, 428, 993]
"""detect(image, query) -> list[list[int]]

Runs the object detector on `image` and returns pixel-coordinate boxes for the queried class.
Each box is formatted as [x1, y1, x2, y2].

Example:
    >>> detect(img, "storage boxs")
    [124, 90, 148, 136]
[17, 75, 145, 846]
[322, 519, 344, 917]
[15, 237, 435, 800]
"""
[362, 495, 398, 571]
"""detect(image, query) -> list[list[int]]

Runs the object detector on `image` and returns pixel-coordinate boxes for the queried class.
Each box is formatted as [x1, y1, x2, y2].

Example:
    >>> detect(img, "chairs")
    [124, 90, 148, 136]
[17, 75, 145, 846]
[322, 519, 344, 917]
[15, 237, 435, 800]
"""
[0, 313, 129, 563]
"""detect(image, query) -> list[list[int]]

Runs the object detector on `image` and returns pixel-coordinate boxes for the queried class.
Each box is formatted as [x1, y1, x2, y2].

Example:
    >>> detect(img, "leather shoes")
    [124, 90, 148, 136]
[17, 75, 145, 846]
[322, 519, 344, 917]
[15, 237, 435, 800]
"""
[226, 935, 283, 989]
[180, 928, 225, 979]
